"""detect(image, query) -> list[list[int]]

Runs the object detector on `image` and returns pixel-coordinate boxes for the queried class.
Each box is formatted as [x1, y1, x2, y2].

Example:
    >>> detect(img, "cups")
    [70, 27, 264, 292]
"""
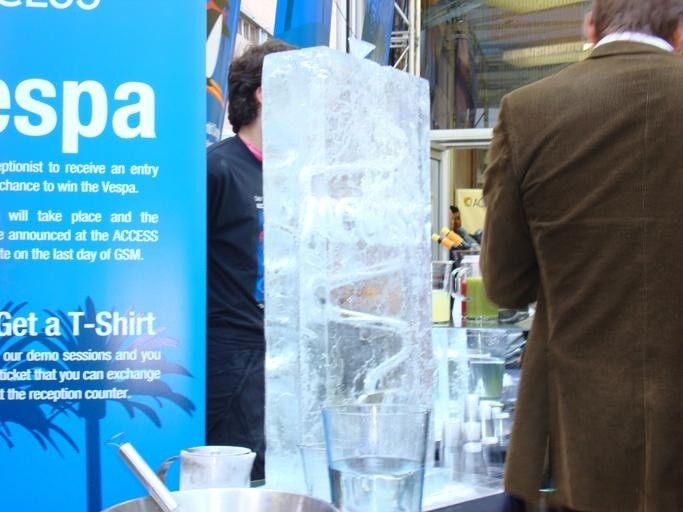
[444, 354, 511, 485]
[156, 445, 257, 489]
[431, 259, 500, 323]
[321, 402, 432, 512]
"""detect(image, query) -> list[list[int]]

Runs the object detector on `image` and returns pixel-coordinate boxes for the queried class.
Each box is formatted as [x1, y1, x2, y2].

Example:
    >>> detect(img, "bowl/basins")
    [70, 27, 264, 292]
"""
[104, 489, 338, 512]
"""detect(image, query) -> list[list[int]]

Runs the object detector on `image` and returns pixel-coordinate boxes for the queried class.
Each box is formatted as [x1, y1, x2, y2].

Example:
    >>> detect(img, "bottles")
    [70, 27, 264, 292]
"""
[433, 227, 479, 257]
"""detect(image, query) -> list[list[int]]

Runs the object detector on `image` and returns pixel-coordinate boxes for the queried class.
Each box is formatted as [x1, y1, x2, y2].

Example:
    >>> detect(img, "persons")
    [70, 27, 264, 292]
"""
[208, 39, 309, 482]
[449, 208, 455, 231]
[449, 205, 478, 248]
[479, 0, 683, 512]
